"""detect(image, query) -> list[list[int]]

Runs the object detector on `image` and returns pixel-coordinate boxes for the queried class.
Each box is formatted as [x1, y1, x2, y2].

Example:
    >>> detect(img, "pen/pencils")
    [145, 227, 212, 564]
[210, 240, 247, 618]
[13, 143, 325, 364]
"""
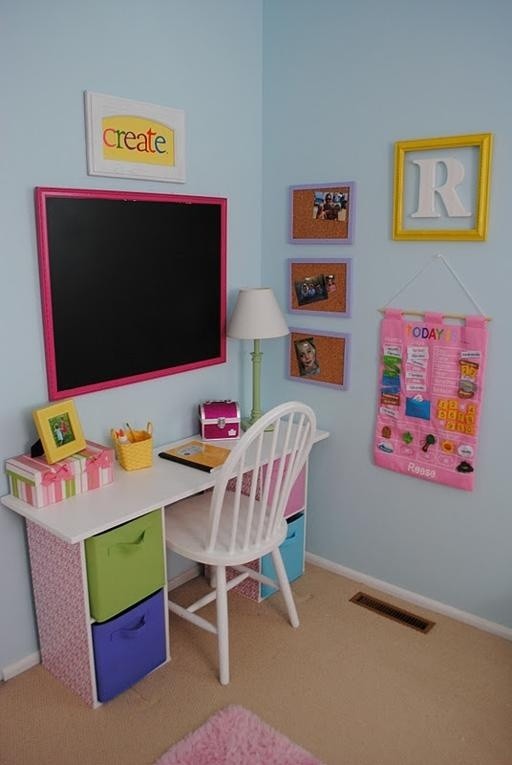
[126, 423, 137, 442]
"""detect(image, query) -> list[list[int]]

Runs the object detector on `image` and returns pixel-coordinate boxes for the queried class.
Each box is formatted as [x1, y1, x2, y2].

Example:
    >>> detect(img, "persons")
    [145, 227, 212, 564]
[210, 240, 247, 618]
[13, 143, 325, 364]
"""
[299, 277, 337, 300]
[53, 423, 65, 446]
[60, 415, 68, 436]
[297, 341, 320, 376]
[314, 190, 347, 220]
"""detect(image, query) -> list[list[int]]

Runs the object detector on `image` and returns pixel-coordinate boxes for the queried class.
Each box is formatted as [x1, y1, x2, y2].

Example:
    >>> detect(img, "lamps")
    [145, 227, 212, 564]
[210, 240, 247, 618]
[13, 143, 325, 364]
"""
[227, 285, 290, 435]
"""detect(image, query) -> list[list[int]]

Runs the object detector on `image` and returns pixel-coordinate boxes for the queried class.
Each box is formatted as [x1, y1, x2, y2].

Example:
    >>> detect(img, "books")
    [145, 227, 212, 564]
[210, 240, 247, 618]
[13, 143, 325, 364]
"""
[158, 440, 232, 474]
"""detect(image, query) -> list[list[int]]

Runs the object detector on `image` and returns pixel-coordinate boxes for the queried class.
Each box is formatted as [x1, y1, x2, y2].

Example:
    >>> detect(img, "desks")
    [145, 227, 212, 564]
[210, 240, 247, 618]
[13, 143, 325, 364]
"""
[4, 415, 333, 714]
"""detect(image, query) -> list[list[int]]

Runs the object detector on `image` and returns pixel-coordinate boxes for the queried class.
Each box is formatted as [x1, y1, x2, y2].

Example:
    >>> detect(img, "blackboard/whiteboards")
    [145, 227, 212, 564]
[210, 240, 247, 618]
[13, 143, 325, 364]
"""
[34, 186, 227, 403]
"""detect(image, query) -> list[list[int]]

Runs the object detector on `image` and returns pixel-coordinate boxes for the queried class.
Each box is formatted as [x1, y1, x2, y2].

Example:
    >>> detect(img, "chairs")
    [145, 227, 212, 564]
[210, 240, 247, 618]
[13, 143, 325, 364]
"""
[163, 399, 318, 686]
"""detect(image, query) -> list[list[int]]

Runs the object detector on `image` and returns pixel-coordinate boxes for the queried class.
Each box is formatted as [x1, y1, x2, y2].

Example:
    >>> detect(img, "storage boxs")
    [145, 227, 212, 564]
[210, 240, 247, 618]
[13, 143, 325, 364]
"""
[197, 400, 241, 441]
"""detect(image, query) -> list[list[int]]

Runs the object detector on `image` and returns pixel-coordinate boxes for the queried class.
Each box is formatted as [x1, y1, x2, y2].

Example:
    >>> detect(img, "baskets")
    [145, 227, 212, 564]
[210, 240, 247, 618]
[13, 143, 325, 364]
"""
[111, 430, 154, 472]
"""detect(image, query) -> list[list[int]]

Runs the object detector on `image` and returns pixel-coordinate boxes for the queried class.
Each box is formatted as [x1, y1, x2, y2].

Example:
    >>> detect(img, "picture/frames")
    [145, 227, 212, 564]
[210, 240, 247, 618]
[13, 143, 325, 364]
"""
[81, 88, 194, 184]
[33, 398, 89, 466]
[281, 176, 358, 391]
[388, 132, 495, 247]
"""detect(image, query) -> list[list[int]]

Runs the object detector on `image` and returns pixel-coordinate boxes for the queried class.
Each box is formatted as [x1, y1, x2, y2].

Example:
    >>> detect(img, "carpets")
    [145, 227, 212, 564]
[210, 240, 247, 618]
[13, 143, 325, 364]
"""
[147, 703, 333, 764]
